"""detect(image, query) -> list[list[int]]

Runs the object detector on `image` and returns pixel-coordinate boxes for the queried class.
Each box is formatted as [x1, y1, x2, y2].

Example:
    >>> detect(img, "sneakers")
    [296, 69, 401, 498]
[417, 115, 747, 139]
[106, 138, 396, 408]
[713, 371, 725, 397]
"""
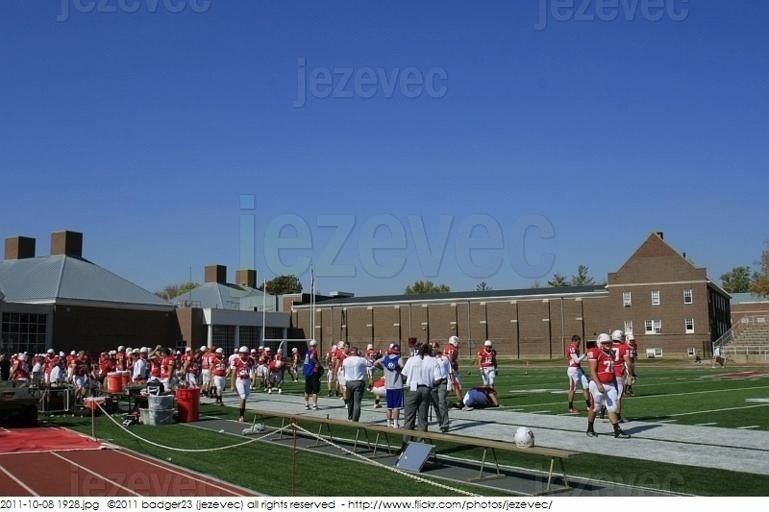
[201, 380, 300, 405]
[326, 391, 348, 408]
[441, 427, 449, 433]
[585, 429, 598, 438]
[600, 409, 605, 419]
[238, 415, 249, 422]
[374, 403, 382, 408]
[613, 426, 632, 439]
[618, 419, 624, 422]
[386, 419, 393, 428]
[312, 406, 320, 410]
[394, 421, 404, 428]
[568, 408, 578, 413]
[622, 390, 635, 397]
[304, 405, 312, 410]
[461, 405, 475, 412]
[587, 406, 590, 412]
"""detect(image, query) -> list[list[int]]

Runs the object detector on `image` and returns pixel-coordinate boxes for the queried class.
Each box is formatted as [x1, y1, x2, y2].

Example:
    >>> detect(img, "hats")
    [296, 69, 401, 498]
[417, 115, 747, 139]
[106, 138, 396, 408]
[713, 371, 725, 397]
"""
[390, 344, 401, 353]
[484, 340, 492, 346]
[309, 339, 319, 347]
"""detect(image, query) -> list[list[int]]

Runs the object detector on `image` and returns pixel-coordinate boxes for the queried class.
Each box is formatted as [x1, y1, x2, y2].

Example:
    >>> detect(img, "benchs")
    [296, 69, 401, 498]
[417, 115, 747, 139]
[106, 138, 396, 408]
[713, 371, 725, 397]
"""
[717, 319, 768, 357]
[246, 403, 585, 495]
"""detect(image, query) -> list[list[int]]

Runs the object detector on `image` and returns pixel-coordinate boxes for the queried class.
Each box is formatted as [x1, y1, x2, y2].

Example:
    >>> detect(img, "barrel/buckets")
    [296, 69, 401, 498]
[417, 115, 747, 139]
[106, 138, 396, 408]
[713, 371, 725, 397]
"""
[105, 371, 132, 394]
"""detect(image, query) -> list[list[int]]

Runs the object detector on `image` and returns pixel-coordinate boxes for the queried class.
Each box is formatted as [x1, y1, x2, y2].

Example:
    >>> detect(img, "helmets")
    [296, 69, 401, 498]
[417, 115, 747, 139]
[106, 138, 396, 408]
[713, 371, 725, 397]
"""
[447, 335, 461, 347]
[612, 330, 625, 343]
[367, 343, 373, 350]
[16, 345, 299, 361]
[596, 332, 613, 350]
[337, 340, 345, 350]
[626, 334, 636, 344]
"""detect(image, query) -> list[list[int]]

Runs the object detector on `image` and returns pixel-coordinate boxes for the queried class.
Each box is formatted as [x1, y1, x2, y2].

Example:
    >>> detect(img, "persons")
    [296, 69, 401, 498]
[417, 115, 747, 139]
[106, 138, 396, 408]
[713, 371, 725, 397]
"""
[565, 329, 638, 439]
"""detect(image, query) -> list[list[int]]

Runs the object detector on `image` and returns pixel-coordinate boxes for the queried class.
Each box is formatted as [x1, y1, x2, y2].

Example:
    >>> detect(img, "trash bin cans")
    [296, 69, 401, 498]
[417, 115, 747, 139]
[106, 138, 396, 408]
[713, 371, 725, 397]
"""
[687, 348, 697, 361]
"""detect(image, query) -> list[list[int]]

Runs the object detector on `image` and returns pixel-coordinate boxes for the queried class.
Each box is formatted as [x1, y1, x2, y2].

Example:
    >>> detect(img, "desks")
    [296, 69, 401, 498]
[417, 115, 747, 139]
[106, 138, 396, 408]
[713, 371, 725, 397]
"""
[92, 387, 173, 418]
